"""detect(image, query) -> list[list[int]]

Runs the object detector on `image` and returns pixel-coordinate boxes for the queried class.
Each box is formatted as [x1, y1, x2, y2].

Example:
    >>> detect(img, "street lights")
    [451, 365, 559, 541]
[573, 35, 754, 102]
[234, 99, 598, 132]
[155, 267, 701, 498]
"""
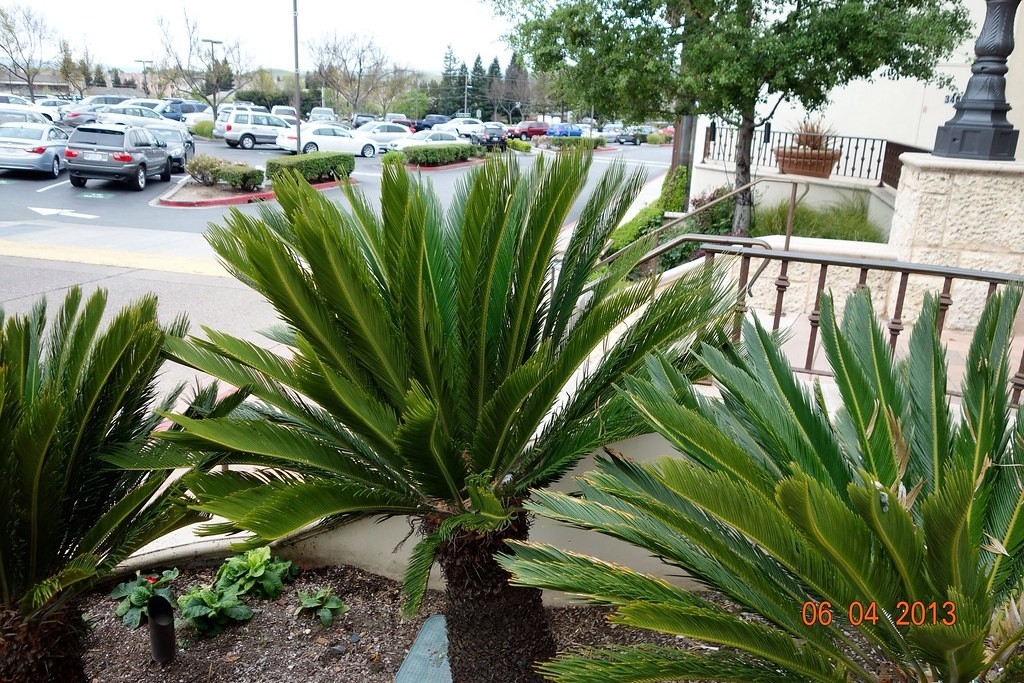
[202, 39, 223, 121]
[136, 60, 154, 97]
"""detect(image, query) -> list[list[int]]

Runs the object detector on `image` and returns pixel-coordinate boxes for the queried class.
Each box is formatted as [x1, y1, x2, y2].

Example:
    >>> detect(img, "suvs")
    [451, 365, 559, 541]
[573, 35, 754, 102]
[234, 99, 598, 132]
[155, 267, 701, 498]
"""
[63, 123, 173, 191]
[507, 120, 550, 141]
[470, 125, 507, 153]
[547, 123, 583, 137]
[433, 117, 483, 137]
[212, 110, 294, 149]
[354, 121, 413, 152]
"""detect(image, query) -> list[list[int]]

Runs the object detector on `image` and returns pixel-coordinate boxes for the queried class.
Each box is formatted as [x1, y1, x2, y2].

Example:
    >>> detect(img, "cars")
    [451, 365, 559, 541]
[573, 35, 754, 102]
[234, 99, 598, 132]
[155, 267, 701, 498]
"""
[576, 123, 675, 146]
[0, 122, 70, 178]
[386, 130, 471, 153]
[276, 123, 380, 158]
[0, 91, 450, 132]
[485, 121, 508, 133]
[143, 124, 195, 174]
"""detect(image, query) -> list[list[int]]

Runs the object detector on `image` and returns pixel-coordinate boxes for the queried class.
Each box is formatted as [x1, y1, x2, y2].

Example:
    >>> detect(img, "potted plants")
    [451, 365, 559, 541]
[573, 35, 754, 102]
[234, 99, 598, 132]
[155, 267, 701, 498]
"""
[773, 120, 842, 178]
